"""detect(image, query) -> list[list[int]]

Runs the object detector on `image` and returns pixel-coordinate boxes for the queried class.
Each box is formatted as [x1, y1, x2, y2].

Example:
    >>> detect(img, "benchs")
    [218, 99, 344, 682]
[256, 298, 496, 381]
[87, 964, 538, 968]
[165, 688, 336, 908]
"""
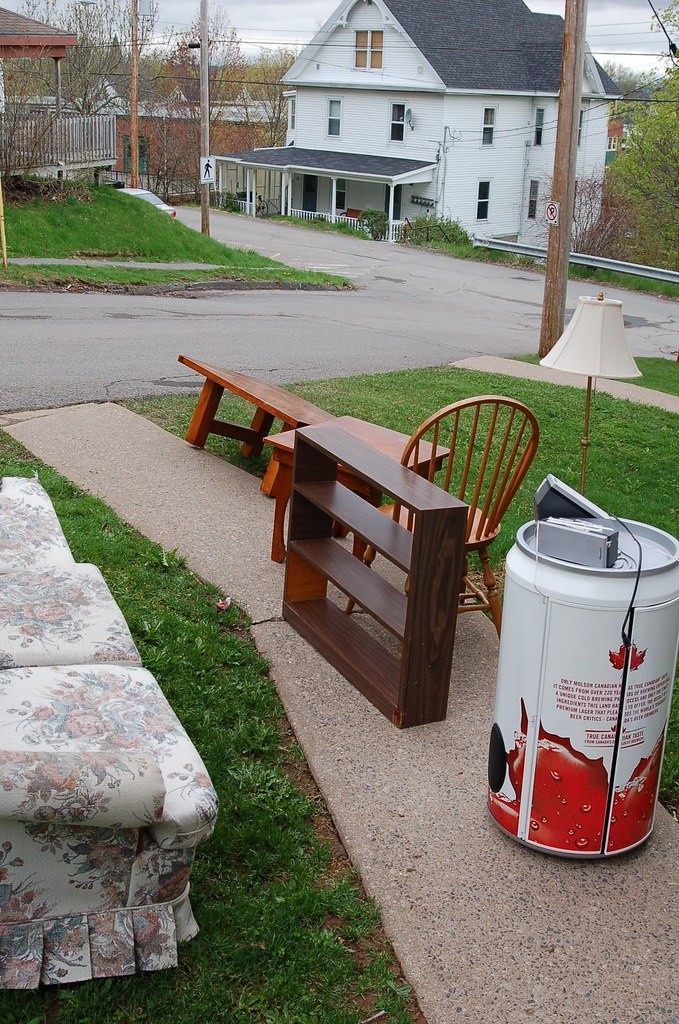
[340, 208, 363, 219]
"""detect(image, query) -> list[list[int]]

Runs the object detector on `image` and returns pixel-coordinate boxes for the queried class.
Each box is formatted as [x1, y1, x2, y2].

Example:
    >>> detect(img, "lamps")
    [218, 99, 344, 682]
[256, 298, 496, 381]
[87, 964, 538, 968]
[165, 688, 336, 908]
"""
[541, 291, 643, 496]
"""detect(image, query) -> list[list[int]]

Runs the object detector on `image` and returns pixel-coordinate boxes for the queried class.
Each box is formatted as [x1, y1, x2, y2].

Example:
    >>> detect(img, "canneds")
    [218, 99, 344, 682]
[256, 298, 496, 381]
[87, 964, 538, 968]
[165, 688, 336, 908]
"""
[485, 514, 678, 858]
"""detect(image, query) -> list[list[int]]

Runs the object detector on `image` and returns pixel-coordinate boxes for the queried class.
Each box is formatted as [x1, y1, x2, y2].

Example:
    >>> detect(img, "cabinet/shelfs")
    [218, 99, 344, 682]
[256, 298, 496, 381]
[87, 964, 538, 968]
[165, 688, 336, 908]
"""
[281, 427, 468, 730]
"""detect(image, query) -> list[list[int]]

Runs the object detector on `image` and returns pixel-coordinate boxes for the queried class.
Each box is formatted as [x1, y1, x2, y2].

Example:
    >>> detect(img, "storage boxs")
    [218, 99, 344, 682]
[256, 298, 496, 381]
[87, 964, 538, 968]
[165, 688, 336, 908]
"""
[533, 473, 611, 519]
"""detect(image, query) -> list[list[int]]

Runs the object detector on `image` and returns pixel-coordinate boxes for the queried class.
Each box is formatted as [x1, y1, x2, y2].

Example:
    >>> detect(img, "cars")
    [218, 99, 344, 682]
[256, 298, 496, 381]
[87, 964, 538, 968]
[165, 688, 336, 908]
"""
[116, 188, 177, 219]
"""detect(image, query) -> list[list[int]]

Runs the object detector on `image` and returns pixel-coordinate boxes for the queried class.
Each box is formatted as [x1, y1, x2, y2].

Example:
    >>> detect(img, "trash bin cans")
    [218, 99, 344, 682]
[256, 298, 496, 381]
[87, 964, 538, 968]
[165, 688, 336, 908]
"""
[105, 180, 125, 189]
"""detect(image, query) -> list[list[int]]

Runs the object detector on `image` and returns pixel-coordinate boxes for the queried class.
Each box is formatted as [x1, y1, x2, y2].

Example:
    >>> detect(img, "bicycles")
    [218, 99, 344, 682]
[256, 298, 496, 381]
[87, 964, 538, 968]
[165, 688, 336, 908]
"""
[256, 195, 268, 215]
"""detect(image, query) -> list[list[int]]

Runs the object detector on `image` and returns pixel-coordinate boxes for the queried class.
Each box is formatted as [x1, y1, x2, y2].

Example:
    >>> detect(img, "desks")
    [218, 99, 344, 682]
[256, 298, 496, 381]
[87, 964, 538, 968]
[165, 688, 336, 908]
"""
[176, 354, 335, 461]
[262, 416, 452, 562]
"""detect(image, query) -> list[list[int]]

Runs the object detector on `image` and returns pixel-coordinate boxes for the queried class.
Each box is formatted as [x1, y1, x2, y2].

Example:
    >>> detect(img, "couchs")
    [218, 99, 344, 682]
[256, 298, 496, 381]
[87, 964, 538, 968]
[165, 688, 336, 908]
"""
[0, 468, 220, 991]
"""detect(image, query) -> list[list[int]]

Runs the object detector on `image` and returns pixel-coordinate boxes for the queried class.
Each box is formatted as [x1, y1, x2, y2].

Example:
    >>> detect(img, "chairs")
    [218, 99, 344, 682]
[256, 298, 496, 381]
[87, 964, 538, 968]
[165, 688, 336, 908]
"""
[347, 394, 543, 640]
